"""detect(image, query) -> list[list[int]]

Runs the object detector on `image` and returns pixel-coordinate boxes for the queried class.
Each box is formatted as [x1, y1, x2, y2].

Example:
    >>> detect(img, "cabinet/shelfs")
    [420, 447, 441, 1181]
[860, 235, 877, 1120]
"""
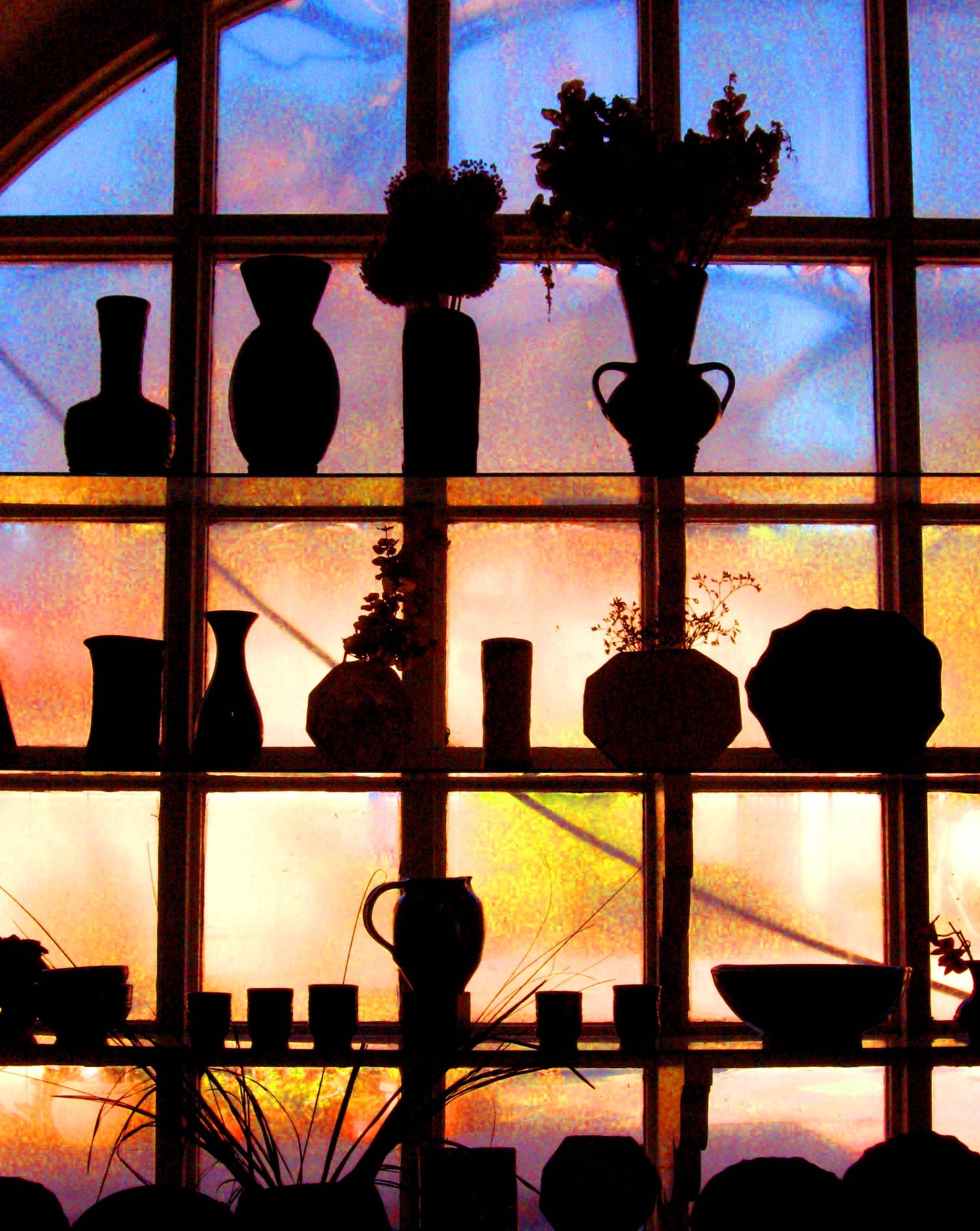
[0, 0, 980, 1231]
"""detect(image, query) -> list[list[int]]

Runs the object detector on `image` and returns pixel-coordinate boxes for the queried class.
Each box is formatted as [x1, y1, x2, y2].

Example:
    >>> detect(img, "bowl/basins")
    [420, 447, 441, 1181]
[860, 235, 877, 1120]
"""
[712, 963, 913, 1054]
[32, 964, 134, 1049]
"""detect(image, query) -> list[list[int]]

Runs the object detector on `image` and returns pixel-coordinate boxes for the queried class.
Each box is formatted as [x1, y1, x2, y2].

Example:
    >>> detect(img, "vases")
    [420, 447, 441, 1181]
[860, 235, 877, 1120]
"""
[591, 266, 737, 476]
[192, 609, 266, 774]
[233, 1179, 394, 1231]
[229, 251, 341, 481]
[478, 635, 533, 775]
[83, 633, 164, 776]
[303, 661, 410, 755]
[582, 647, 742, 776]
[398, 307, 482, 479]
[63, 292, 178, 481]
[361, 873, 487, 1055]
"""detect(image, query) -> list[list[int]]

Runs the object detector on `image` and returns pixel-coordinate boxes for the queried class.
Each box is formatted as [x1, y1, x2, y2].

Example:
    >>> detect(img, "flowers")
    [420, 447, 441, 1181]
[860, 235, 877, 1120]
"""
[357, 156, 509, 315]
[518, 68, 800, 277]
[336, 515, 454, 677]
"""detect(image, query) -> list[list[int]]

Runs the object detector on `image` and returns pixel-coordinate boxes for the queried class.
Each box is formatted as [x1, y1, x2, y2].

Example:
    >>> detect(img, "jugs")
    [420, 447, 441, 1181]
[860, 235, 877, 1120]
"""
[363, 875, 485, 994]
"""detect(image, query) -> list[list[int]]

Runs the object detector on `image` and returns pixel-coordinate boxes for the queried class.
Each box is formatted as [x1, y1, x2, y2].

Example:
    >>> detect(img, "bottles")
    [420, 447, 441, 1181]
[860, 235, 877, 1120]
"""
[193, 610, 264, 772]
[62, 294, 174, 478]
[227, 254, 341, 475]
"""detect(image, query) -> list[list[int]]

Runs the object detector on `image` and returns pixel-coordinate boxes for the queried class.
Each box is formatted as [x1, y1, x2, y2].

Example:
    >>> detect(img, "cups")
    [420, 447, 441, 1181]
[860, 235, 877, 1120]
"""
[309, 985, 359, 1048]
[82, 634, 164, 770]
[247, 988, 294, 1048]
[536, 991, 582, 1050]
[614, 984, 660, 1048]
[417, 1145, 519, 1231]
[482, 637, 533, 772]
[187, 991, 232, 1048]
